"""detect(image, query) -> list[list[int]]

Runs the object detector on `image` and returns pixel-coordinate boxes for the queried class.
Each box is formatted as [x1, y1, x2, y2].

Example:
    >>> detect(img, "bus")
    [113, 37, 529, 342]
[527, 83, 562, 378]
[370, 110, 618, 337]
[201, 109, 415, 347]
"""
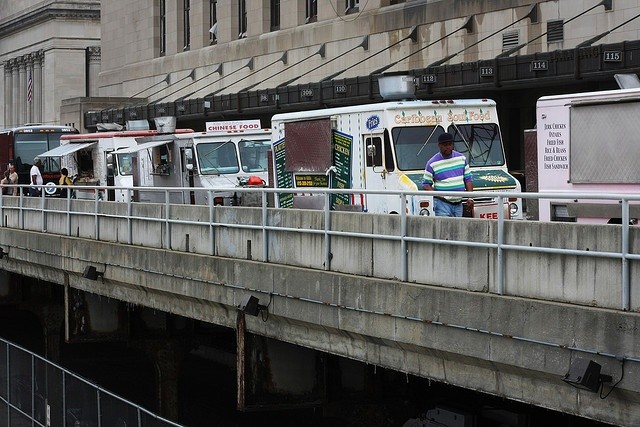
[0, 123, 80, 195]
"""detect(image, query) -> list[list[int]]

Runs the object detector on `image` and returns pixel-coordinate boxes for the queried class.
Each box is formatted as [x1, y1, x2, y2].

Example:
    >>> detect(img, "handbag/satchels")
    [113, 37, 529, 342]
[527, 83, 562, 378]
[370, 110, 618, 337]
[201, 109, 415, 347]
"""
[61, 177, 73, 198]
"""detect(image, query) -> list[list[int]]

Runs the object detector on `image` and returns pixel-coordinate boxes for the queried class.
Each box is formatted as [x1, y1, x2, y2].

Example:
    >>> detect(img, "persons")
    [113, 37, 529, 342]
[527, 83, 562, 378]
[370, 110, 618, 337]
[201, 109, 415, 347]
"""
[1, 161, 18, 196]
[421, 133, 474, 217]
[59, 168, 74, 198]
[29, 157, 43, 197]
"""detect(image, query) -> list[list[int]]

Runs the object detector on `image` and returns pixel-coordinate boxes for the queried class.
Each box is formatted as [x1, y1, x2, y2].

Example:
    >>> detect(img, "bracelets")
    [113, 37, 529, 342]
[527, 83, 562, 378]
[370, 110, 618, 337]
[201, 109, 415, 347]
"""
[468, 197, 474, 201]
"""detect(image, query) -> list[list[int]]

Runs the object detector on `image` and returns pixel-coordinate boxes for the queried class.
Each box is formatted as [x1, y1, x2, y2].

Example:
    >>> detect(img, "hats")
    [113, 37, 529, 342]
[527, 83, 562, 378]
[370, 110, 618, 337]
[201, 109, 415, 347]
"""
[438, 134, 454, 144]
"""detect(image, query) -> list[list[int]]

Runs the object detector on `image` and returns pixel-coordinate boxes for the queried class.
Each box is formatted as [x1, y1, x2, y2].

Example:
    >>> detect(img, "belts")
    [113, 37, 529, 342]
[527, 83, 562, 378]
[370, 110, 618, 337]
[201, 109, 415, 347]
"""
[433, 196, 462, 206]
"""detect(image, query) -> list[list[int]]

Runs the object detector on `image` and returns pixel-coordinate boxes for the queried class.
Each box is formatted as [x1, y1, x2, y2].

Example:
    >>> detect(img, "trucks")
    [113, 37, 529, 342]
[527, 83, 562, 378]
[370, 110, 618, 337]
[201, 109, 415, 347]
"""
[272, 99, 522, 219]
[536, 88, 640, 224]
[111, 129, 271, 206]
[38, 128, 194, 201]
